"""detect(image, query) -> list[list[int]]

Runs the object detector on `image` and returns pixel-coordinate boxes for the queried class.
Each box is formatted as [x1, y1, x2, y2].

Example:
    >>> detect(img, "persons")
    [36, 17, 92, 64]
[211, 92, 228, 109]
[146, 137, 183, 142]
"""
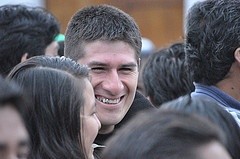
[0, 0, 240, 159]
